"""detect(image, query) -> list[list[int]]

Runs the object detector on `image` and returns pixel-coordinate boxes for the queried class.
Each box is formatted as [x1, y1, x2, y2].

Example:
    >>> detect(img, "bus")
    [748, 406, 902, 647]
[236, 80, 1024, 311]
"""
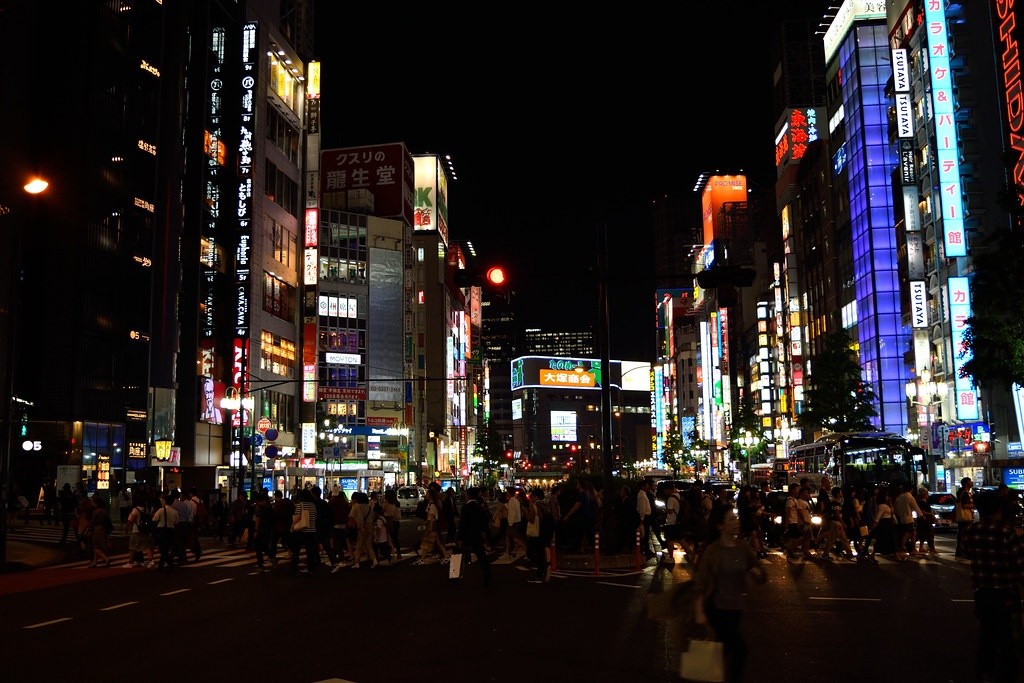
[750, 456, 786, 490]
[786, 430, 928, 542]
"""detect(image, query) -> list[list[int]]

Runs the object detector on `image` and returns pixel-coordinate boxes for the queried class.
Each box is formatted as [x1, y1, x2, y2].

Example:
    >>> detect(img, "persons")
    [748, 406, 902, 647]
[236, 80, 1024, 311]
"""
[615, 484, 643, 554]
[887, 464, 908, 481]
[210, 484, 402, 576]
[6, 484, 20, 530]
[412, 481, 606, 584]
[955, 479, 1024, 683]
[52, 481, 207, 567]
[735, 472, 789, 559]
[634, 474, 733, 564]
[782, 478, 944, 564]
[686, 505, 770, 683]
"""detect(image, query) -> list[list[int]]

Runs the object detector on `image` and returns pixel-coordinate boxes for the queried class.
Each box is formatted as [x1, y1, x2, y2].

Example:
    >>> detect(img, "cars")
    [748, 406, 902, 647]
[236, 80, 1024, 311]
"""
[762, 490, 828, 545]
[928, 491, 963, 532]
[654, 480, 740, 518]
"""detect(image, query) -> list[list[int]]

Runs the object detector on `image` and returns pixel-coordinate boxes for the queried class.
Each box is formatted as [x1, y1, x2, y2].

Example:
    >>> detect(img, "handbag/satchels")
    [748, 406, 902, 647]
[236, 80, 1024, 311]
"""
[448, 547, 463, 578]
[859, 525, 870, 538]
[292, 503, 310, 531]
[525, 504, 539, 539]
[103, 516, 114, 534]
[240, 527, 249, 543]
[679, 623, 724, 682]
[429, 502, 448, 534]
[155, 527, 169, 545]
[348, 519, 356, 527]
[954, 491, 972, 522]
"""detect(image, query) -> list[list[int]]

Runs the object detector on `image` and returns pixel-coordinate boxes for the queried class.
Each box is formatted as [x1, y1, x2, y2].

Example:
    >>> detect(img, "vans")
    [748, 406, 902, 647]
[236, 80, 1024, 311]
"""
[397, 485, 425, 517]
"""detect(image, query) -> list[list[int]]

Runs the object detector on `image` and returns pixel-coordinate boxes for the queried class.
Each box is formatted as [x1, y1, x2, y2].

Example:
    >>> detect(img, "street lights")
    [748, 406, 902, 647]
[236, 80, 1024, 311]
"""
[334, 434, 347, 476]
[739, 431, 759, 490]
[690, 447, 707, 479]
[221, 387, 255, 503]
[906, 365, 949, 491]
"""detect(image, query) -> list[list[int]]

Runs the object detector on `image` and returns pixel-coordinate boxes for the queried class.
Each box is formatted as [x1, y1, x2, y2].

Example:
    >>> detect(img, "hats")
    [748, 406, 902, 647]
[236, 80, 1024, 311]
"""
[240, 491, 248, 498]
[692, 481, 703, 491]
[646, 478, 655, 483]
[920, 488, 928, 493]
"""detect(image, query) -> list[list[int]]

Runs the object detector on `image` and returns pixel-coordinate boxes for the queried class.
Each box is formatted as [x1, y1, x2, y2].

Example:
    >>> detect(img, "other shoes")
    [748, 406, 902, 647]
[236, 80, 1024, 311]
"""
[643, 542, 946, 561]
[55, 538, 542, 573]
[527, 576, 543, 582]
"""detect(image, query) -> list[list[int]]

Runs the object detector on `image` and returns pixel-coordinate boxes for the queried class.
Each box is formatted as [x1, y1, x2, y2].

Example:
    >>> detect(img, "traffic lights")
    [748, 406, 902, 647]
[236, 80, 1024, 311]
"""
[19, 412, 30, 437]
[457, 269, 504, 286]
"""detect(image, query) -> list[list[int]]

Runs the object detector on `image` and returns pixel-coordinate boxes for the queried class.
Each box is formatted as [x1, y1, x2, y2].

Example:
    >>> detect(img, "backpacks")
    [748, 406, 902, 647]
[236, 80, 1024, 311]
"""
[191, 497, 209, 530]
[132, 507, 154, 536]
[666, 495, 689, 527]
